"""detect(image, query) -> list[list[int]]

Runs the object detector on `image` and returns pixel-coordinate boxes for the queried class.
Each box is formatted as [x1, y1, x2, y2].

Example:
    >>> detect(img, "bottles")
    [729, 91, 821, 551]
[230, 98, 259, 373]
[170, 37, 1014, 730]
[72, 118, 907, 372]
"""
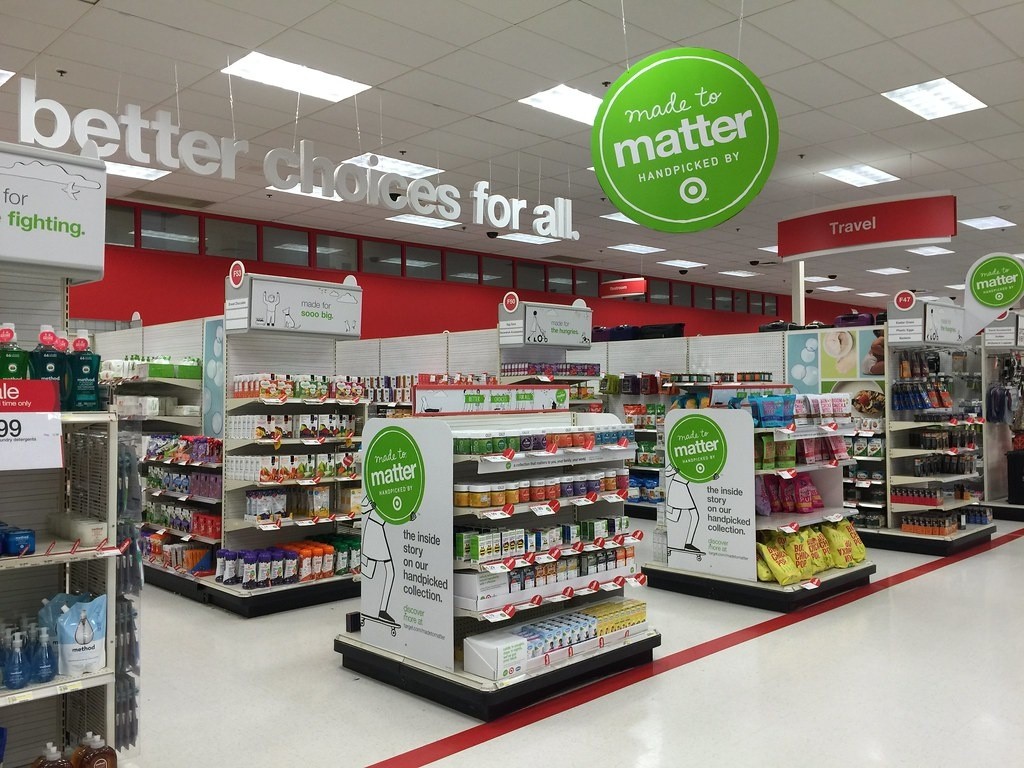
[0, 323, 101, 411]
[215, 532, 362, 590]
[35, 732, 118, 768]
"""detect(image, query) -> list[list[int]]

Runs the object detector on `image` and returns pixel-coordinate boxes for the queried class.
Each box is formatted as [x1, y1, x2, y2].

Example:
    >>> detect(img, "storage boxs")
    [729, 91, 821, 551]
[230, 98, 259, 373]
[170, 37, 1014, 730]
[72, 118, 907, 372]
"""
[464, 599, 646, 681]
[521, 567, 535, 590]
[555, 558, 567, 584]
[112, 394, 201, 418]
[525, 533, 536, 552]
[482, 534, 493, 562]
[514, 528, 525, 555]
[490, 533, 502, 560]
[537, 530, 550, 551]
[549, 528, 557, 548]
[456, 531, 477, 562]
[558, 513, 630, 545]
[454, 569, 507, 599]
[534, 565, 547, 588]
[587, 554, 597, 575]
[499, 531, 509, 559]
[506, 530, 516, 557]
[578, 554, 588, 577]
[544, 562, 557, 585]
[470, 534, 486, 564]
[564, 557, 578, 580]
[509, 569, 522, 593]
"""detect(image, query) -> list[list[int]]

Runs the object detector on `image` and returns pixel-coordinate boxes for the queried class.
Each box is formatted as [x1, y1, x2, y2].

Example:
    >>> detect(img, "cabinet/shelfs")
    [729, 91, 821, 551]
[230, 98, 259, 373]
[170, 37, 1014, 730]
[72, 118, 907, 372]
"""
[962, 310, 1024, 520]
[639, 394, 875, 615]
[337, 291, 602, 415]
[565, 298, 1024, 556]
[0, 136, 145, 768]
[334, 390, 665, 718]
[86, 261, 373, 619]
